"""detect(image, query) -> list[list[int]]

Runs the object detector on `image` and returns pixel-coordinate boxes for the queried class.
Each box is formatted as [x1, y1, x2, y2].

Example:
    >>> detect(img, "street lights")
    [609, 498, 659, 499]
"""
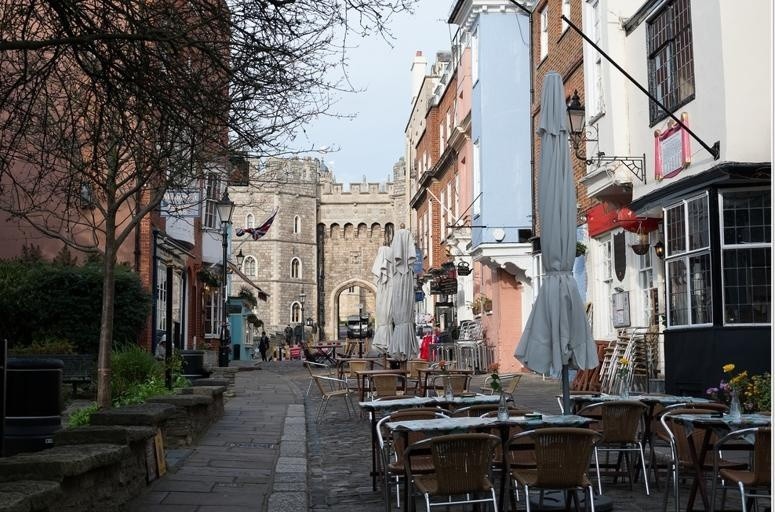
[299, 283, 305, 347]
[297, 287, 308, 342]
[356, 302, 365, 360]
[236, 249, 245, 270]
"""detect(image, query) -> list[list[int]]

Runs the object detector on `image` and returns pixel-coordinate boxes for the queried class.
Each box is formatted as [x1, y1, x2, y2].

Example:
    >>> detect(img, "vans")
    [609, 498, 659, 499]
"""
[345, 315, 373, 339]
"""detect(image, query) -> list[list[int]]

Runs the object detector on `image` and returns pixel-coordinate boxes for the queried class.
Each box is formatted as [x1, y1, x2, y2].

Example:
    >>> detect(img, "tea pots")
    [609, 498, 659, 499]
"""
[457, 261, 474, 276]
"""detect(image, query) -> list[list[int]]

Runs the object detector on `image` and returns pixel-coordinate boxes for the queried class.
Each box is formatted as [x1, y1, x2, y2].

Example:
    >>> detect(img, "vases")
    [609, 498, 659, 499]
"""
[446, 376, 454, 401]
[498, 391, 510, 421]
[730, 390, 742, 420]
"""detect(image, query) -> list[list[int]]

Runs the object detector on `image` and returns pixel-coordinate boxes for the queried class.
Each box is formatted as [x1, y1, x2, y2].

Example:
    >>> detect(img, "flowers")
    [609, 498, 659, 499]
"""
[438, 359, 453, 393]
[488, 363, 505, 411]
[707, 372, 771, 413]
[723, 364, 736, 391]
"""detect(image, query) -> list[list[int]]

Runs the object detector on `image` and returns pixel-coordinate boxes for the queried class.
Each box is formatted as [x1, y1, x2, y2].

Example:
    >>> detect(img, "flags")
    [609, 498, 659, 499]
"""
[235, 208, 277, 241]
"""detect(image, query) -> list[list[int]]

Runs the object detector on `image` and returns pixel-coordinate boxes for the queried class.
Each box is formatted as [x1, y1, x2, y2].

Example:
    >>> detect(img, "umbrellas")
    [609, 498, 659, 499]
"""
[515, 71, 600, 503]
[371, 242, 394, 369]
[388, 224, 418, 390]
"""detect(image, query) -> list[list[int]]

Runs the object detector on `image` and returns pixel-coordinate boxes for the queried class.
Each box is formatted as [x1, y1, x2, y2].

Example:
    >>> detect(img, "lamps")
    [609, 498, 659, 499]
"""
[562, 90, 601, 165]
[653, 239, 665, 261]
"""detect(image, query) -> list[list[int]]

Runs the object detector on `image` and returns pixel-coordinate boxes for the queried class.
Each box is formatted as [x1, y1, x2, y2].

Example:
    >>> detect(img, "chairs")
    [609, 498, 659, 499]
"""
[660, 410, 748, 512]
[369, 374, 407, 402]
[345, 361, 369, 399]
[646, 402, 728, 497]
[710, 429, 771, 511]
[369, 396, 419, 423]
[451, 405, 520, 417]
[453, 319, 490, 375]
[303, 361, 336, 397]
[555, 392, 626, 477]
[401, 434, 501, 512]
[479, 411, 534, 502]
[433, 375, 472, 398]
[390, 408, 453, 417]
[574, 401, 650, 497]
[376, 412, 451, 512]
[408, 362, 429, 398]
[505, 427, 604, 512]
[600, 324, 659, 395]
[312, 374, 356, 425]
[480, 376, 505, 395]
[504, 375, 521, 407]
[299, 340, 357, 366]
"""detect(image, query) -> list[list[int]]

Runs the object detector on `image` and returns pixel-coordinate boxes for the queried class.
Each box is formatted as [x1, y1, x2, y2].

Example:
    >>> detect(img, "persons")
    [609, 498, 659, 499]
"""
[155, 334, 166, 361]
[284, 324, 293, 346]
[418, 325, 423, 338]
[258, 331, 269, 362]
[295, 322, 301, 344]
